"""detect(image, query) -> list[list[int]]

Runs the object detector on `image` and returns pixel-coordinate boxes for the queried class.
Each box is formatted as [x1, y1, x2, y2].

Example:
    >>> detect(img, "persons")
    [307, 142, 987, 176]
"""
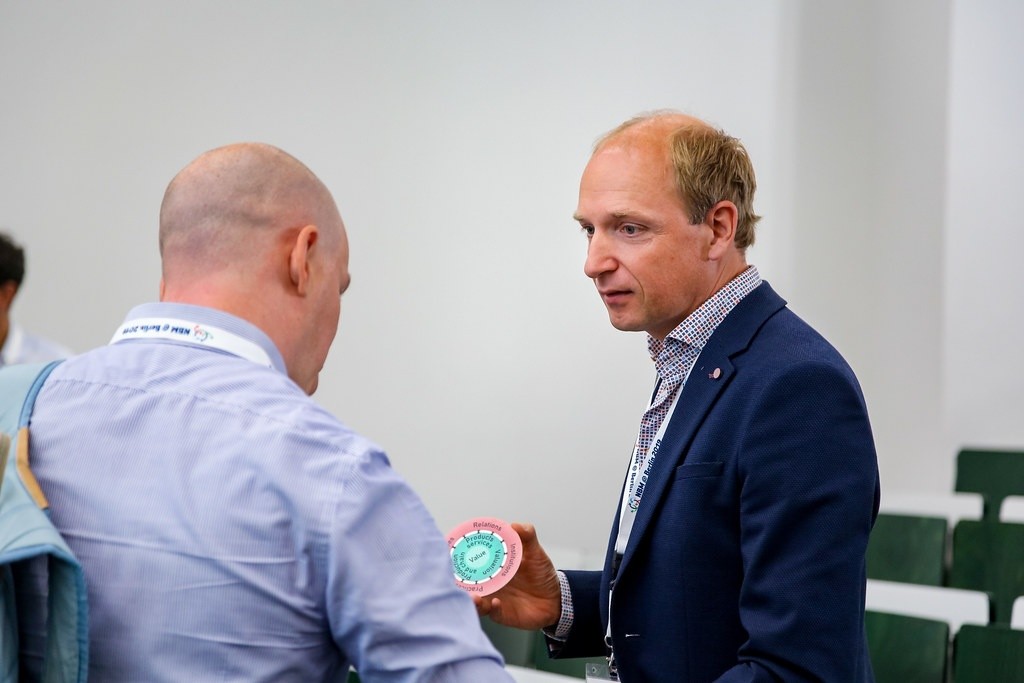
[0, 235, 69, 364]
[0, 146, 514, 683]
[470, 110, 880, 683]
